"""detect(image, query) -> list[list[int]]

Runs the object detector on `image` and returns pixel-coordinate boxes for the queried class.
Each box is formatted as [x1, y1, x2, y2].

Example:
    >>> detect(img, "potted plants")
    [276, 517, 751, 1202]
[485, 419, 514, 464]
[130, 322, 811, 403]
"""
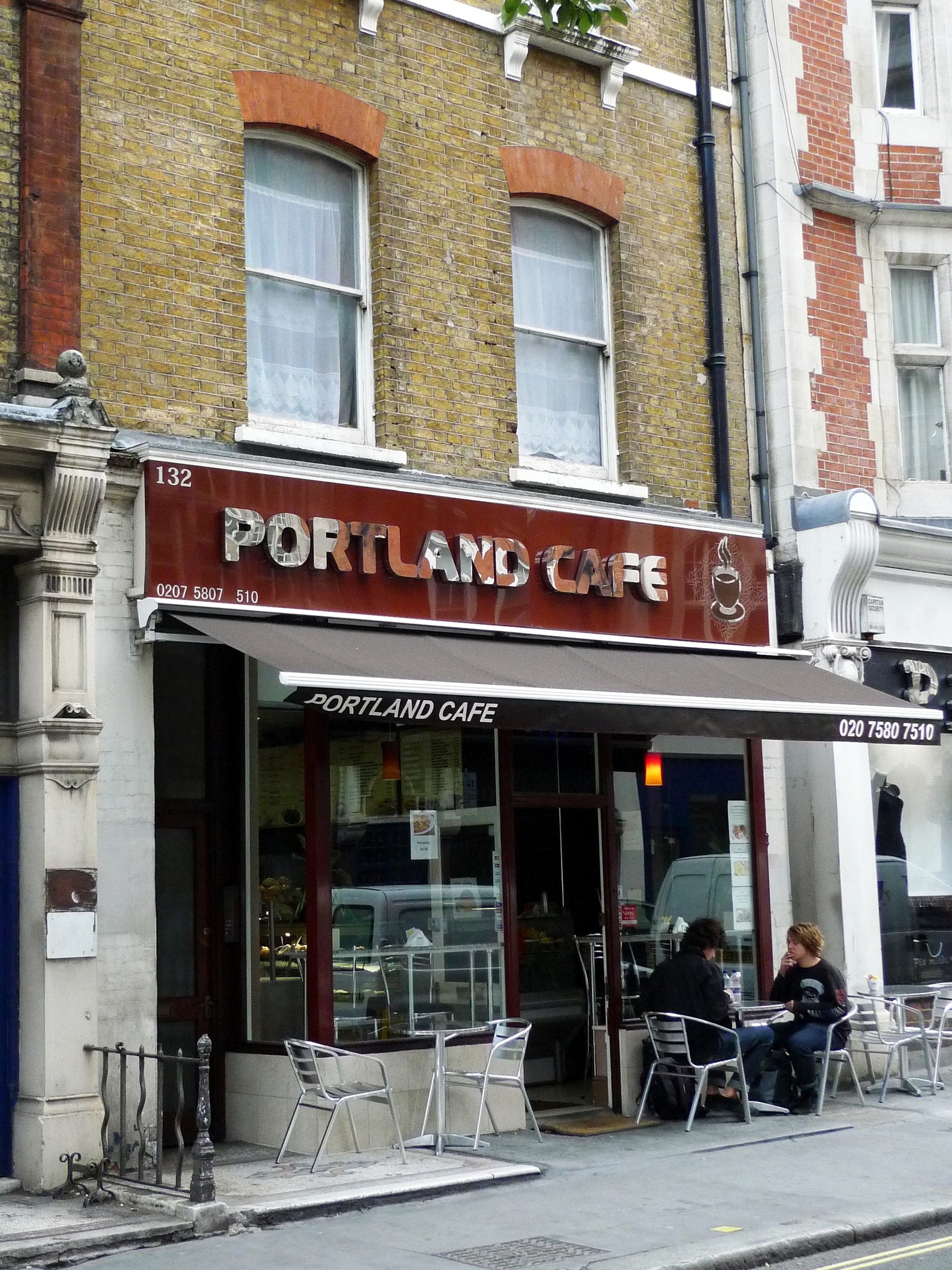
[660, 915, 674, 932]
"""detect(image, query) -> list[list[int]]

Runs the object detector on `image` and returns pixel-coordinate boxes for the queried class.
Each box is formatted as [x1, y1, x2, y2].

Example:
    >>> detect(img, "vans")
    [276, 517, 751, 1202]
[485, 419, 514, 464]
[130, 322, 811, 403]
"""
[332, 885, 500, 1019]
[618, 855, 952, 1003]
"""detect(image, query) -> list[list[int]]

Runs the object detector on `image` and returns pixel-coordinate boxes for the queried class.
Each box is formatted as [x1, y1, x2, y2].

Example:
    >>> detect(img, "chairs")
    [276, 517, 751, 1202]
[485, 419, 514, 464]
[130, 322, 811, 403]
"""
[636, 1011, 751, 1134]
[882, 982, 952, 1094]
[279, 1034, 408, 1173]
[421, 1018, 543, 1151]
[811, 998, 866, 1116]
[830, 994, 937, 1104]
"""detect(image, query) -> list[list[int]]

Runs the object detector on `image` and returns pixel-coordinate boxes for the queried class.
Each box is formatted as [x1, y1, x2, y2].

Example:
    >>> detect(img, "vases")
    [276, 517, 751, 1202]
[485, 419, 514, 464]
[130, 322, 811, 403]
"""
[868, 980, 878, 990]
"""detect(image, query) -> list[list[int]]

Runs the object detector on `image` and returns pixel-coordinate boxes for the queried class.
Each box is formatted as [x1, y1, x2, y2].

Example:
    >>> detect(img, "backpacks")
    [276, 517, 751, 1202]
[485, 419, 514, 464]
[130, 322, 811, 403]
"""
[635, 1036, 710, 1120]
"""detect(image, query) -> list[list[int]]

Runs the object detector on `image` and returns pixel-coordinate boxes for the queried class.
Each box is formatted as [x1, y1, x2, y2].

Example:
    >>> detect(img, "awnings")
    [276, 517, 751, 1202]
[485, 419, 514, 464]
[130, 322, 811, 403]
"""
[169, 612, 945, 750]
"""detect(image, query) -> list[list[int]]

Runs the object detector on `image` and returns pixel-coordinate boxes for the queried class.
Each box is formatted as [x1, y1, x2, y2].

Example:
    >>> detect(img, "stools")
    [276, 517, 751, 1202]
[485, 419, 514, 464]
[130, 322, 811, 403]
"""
[571, 933, 642, 1099]
[626, 930, 672, 986]
[295, 943, 433, 1041]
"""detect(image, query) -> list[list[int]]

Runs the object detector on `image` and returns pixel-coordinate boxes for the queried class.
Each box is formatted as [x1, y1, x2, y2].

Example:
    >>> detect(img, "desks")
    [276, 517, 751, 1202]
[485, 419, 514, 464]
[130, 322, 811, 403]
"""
[387, 1022, 492, 1156]
[728, 1000, 791, 1117]
[281, 943, 503, 1032]
[857, 984, 944, 1098]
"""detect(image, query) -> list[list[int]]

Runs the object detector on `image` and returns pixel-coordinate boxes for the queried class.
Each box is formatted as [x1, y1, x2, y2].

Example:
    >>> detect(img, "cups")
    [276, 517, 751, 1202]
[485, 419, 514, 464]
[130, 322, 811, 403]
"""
[654, 922, 669, 933]
[868, 980, 878, 991]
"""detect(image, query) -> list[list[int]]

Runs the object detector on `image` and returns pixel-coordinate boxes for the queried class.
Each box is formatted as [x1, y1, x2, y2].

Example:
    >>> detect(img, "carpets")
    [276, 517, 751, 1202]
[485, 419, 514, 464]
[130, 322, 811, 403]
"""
[540, 1112, 664, 1137]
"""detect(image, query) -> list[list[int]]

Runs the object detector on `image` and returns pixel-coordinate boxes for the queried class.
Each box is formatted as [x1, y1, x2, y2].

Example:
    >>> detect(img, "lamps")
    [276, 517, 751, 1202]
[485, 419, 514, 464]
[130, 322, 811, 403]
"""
[381, 724, 402, 781]
[644, 737, 663, 786]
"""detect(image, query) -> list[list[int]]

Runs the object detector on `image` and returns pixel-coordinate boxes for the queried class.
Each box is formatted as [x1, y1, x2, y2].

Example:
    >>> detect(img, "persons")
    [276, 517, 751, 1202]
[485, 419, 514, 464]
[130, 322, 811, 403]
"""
[646, 918, 774, 1116]
[769, 922, 852, 1115]
[877, 782, 906, 860]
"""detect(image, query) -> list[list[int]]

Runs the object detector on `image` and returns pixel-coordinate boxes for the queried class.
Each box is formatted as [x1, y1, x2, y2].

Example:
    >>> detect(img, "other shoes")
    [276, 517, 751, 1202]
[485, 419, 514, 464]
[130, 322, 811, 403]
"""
[704, 1092, 760, 1116]
[793, 1090, 819, 1115]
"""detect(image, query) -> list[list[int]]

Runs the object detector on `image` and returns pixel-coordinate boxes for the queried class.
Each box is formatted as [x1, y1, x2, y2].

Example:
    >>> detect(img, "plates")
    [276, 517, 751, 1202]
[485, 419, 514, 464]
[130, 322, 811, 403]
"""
[413, 814, 434, 835]
[282, 808, 302, 825]
[736, 910, 751, 922]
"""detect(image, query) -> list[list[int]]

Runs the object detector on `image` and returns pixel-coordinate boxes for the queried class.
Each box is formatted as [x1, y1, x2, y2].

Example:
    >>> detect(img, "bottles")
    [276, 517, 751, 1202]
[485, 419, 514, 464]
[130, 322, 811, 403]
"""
[730, 970, 742, 1004]
[722, 970, 733, 1006]
[673, 917, 687, 933]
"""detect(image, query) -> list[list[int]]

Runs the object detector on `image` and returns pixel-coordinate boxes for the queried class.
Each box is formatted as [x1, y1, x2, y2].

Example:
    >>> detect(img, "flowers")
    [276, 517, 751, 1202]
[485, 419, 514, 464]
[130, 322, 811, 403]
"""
[865, 974, 878, 981]
